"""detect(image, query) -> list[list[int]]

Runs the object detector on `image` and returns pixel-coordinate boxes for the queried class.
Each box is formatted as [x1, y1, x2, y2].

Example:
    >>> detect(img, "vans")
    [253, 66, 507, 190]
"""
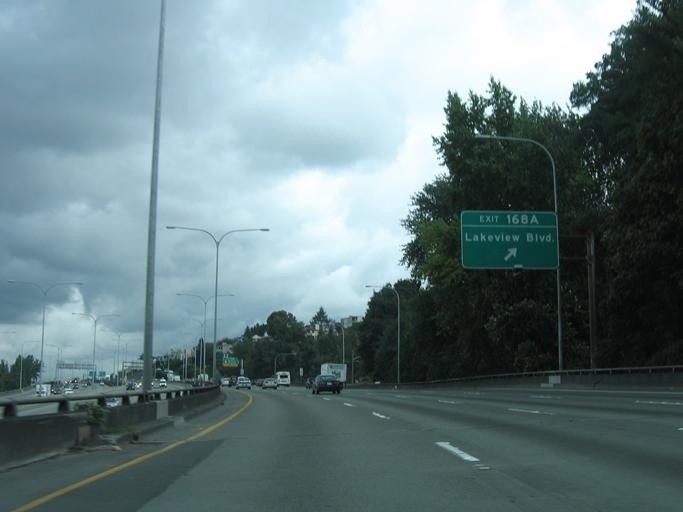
[275, 370, 290, 387]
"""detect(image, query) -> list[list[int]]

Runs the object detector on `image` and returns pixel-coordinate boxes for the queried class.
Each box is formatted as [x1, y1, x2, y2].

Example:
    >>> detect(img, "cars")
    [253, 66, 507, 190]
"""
[51, 378, 90, 396]
[158, 378, 168, 388]
[126, 380, 142, 390]
[306, 374, 343, 396]
[99, 381, 105, 386]
[219, 375, 251, 390]
[254, 378, 277, 389]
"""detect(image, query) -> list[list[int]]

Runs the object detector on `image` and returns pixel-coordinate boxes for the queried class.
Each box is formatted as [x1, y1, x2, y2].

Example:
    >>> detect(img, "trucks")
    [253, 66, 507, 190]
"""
[320, 362, 348, 388]
[34, 384, 52, 398]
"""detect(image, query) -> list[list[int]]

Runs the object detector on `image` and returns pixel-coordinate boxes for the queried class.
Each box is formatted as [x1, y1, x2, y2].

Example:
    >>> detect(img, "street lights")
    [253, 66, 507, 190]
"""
[7, 278, 83, 386]
[471, 134, 563, 371]
[185, 313, 222, 380]
[72, 312, 121, 377]
[166, 225, 269, 381]
[175, 292, 234, 382]
[274, 352, 297, 372]
[365, 284, 400, 387]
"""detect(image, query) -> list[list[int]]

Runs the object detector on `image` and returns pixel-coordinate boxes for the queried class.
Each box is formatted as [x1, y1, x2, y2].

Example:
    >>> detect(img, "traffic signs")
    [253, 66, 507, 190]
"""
[461, 211, 559, 270]
